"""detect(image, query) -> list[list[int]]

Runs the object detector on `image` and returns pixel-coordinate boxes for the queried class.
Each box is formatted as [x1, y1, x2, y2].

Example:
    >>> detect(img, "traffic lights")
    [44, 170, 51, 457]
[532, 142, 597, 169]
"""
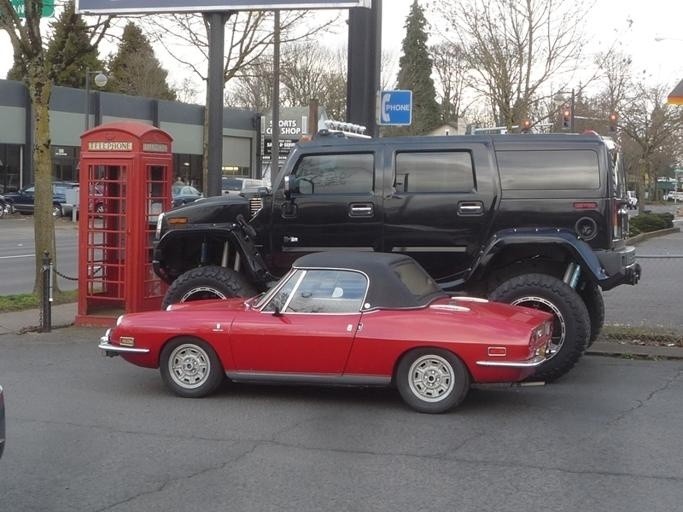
[608, 114, 617, 132]
[523, 119, 530, 131]
[561, 110, 571, 128]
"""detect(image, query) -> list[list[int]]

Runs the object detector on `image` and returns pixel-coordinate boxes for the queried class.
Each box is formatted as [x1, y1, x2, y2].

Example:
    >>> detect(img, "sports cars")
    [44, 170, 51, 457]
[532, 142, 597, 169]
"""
[97, 246, 557, 414]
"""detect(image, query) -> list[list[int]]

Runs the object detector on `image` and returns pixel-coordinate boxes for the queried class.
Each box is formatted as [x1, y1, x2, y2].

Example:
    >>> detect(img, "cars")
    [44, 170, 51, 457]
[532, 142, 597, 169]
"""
[0, 175, 275, 224]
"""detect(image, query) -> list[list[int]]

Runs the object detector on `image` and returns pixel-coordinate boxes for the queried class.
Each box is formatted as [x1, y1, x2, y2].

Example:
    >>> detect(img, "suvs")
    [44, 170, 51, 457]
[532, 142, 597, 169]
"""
[625, 190, 638, 210]
[149, 129, 643, 353]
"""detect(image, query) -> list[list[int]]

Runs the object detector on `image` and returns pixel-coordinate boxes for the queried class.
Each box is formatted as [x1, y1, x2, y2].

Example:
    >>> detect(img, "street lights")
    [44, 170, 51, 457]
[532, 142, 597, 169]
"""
[83, 65, 108, 130]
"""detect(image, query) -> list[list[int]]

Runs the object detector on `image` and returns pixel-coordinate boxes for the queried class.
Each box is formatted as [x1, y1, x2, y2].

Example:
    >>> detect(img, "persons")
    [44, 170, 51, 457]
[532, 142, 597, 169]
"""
[173, 176, 185, 185]
